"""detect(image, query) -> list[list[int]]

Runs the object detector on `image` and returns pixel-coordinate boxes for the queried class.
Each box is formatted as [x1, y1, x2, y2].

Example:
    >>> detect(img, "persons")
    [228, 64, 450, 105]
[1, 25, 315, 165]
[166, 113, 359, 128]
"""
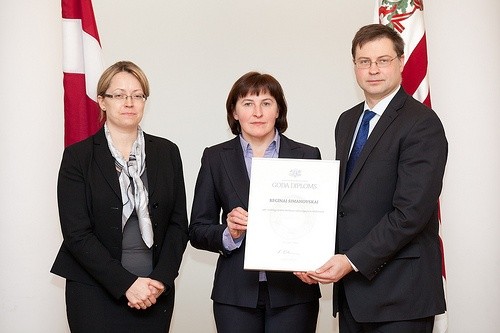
[306, 24, 448, 333]
[50, 61, 189, 333]
[189, 71, 322, 333]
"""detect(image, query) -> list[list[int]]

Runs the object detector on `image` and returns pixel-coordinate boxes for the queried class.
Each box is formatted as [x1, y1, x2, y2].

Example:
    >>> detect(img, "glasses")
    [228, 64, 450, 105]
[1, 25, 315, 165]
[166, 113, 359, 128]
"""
[102, 93, 147, 102]
[354, 55, 398, 69]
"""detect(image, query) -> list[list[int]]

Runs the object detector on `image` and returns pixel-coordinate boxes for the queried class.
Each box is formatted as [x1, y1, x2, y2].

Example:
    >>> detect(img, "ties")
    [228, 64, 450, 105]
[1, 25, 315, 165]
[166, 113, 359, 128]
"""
[343, 109, 376, 191]
[104, 121, 154, 248]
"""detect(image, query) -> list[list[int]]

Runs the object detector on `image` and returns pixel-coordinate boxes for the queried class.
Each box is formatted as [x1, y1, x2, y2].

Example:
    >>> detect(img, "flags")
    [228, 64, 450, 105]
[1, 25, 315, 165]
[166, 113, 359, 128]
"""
[377, 0, 447, 333]
[61, 0, 107, 148]
